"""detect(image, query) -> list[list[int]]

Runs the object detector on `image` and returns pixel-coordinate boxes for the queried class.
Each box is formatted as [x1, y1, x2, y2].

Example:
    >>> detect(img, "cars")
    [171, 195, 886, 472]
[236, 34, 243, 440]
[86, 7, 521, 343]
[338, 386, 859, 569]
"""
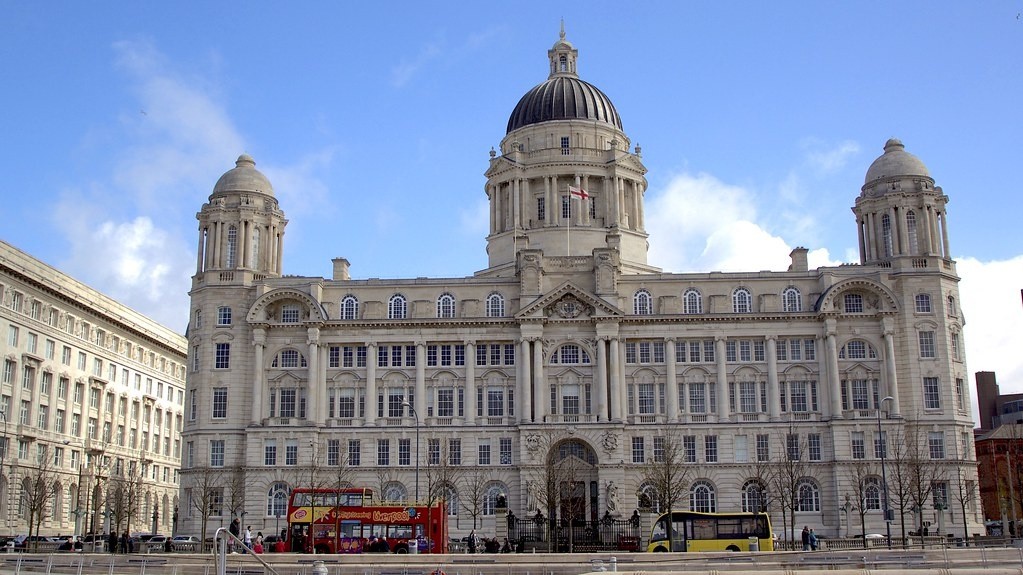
[148, 536, 167, 549]
[173, 535, 199, 550]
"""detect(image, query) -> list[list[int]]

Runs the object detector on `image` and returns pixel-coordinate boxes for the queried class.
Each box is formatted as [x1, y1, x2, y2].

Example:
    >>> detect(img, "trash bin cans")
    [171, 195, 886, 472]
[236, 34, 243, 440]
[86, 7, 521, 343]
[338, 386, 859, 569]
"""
[313, 566, 327, 575]
[95, 540, 104, 553]
[956, 538, 963, 546]
[749, 536, 758, 551]
[408, 539, 418, 554]
[7, 542, 15, 553]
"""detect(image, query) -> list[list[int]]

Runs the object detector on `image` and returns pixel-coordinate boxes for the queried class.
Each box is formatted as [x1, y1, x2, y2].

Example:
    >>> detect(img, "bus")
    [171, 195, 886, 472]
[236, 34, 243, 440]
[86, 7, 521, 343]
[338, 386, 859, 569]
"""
[286, 488, 448, 554]
[646, 511, 773, 553]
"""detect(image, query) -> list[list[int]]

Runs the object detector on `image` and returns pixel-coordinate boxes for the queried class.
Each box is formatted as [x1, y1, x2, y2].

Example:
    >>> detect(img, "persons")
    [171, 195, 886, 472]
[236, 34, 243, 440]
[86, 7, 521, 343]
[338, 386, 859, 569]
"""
[370, 538, 380, 552]
[468, 530, 476, 553]
[268, 538, 285, 553]
[485, 537, 500, 553]
[253, 531, 264, 554]
[164, 537, 175, 552]
[801, 525, 810, 551]
[59, 537, 84, 552]
[229, 517, 240, 538]
[527, 480, 541, 511]
[363, 538, 370, 552]
[244, 525, 253, 549]
[755, 524, 763, 533]
[501, 538, 512, 553]
[379, 537, 391, 552]
[605, 480, 619, 512]
[121, 533, 135, 554]
[108, 531, 118, 553]
[809, 528, 818, 551]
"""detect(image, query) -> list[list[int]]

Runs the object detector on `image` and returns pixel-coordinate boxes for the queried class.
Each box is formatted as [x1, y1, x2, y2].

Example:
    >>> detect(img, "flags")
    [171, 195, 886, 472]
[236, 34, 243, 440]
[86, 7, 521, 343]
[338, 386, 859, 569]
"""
[569, 186, 589, 201]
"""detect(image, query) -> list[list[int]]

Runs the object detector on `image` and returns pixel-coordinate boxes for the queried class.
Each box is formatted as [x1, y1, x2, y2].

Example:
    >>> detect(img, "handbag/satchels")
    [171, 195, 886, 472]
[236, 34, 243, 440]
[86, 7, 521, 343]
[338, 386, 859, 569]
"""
[228, 535, 233, 544]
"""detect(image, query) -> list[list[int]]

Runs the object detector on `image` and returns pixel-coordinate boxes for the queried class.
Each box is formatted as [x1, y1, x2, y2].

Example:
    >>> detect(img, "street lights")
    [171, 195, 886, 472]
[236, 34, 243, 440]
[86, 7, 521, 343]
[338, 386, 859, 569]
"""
[878, 396, 894, 549]
[401, 402, 419, 505]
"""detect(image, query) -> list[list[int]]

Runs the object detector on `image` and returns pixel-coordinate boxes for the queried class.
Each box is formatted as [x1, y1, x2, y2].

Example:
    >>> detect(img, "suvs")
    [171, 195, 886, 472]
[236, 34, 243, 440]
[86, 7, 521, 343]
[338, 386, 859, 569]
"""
[23, 535, 109, 553]
